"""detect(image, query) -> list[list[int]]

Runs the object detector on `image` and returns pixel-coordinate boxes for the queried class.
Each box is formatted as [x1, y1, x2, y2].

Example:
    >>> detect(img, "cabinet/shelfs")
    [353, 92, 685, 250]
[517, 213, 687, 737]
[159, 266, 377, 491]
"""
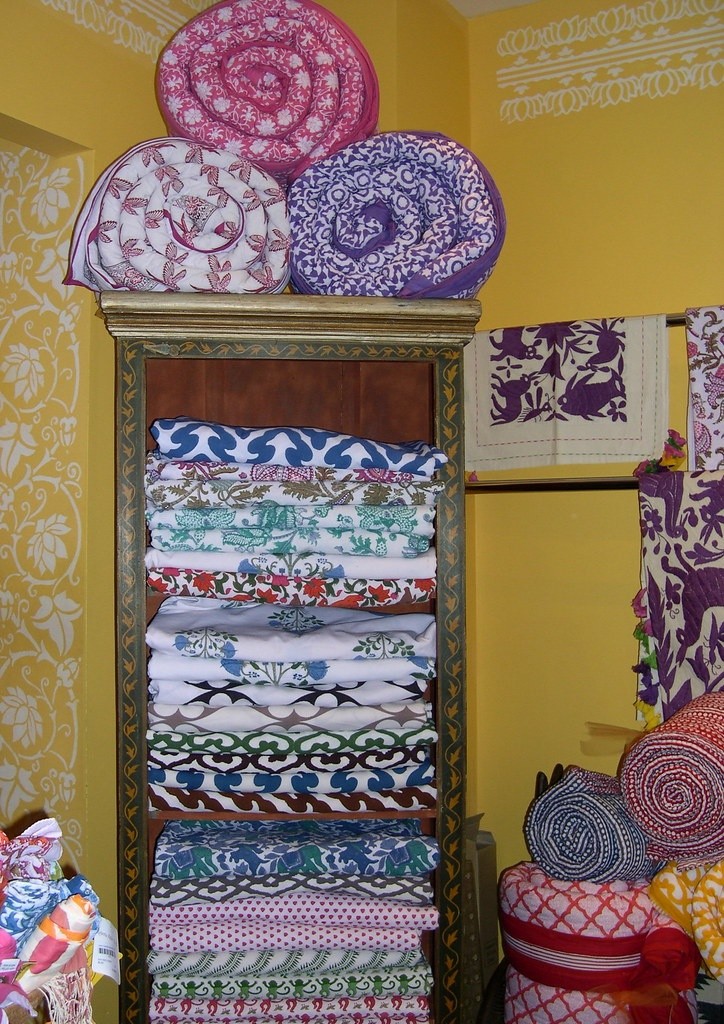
[98, 291, 481, 1024]
